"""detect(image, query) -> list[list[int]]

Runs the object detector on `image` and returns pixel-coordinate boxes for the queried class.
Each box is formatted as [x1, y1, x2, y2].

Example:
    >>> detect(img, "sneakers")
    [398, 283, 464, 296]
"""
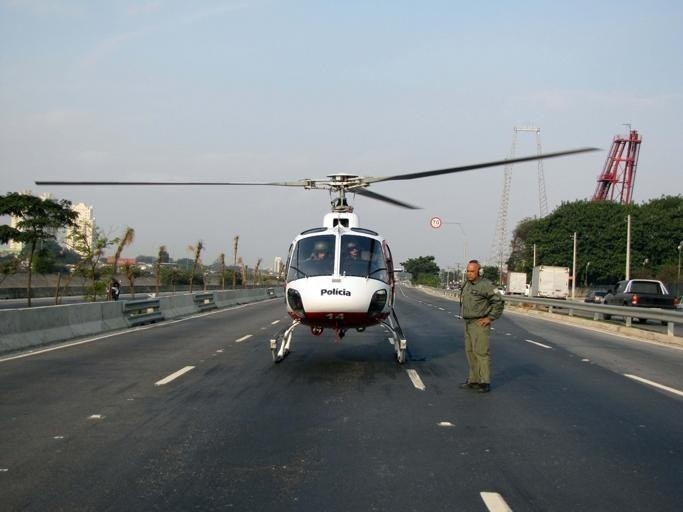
[458, 383, 491, 392]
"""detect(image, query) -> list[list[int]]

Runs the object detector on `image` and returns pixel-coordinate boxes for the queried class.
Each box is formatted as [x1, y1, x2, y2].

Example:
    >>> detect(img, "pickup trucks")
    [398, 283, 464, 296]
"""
[601, 278, 675, 327]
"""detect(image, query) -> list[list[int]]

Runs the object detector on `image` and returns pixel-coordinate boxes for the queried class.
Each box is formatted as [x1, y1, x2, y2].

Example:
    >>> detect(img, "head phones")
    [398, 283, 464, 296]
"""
[464, 268, 484, 276]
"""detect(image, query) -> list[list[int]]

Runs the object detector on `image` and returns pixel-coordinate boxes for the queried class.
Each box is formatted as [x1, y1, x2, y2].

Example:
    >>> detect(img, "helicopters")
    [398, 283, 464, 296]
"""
[32, 140, 606, 366]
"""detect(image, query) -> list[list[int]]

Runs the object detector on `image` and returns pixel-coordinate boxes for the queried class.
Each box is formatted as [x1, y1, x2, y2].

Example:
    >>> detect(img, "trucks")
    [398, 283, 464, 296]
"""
[531, 264, 570, 301]
[504, 271, 526, 296]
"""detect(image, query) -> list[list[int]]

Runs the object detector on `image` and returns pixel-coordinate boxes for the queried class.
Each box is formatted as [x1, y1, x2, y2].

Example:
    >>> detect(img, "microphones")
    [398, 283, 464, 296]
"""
[471, 277, 483, 285]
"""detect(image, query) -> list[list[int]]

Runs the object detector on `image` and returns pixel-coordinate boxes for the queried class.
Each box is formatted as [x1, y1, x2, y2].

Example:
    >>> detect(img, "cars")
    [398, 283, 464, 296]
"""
[444, 277, 531, 296]
[583, 290, 609, 305]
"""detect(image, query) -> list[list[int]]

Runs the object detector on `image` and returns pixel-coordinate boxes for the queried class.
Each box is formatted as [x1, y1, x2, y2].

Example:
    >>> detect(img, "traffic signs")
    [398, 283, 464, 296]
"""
[429, 216, 441, 229]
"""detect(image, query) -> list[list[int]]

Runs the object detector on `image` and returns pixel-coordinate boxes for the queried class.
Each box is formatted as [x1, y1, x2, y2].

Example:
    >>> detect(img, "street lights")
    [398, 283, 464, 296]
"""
[641, 257, 648, 272]
[583, 261, 592, 288]
[676, 241, 682, 297]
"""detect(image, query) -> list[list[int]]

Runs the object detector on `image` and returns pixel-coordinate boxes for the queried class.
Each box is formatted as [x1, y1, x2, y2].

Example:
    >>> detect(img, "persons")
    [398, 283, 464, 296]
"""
[457, 261, 505, 393]
[305, 240, 331, 275]
[346, 242, 363, 271]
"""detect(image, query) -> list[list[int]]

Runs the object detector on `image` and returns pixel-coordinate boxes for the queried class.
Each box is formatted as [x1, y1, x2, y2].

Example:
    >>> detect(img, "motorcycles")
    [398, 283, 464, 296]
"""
[105, 282, 120, 301]
